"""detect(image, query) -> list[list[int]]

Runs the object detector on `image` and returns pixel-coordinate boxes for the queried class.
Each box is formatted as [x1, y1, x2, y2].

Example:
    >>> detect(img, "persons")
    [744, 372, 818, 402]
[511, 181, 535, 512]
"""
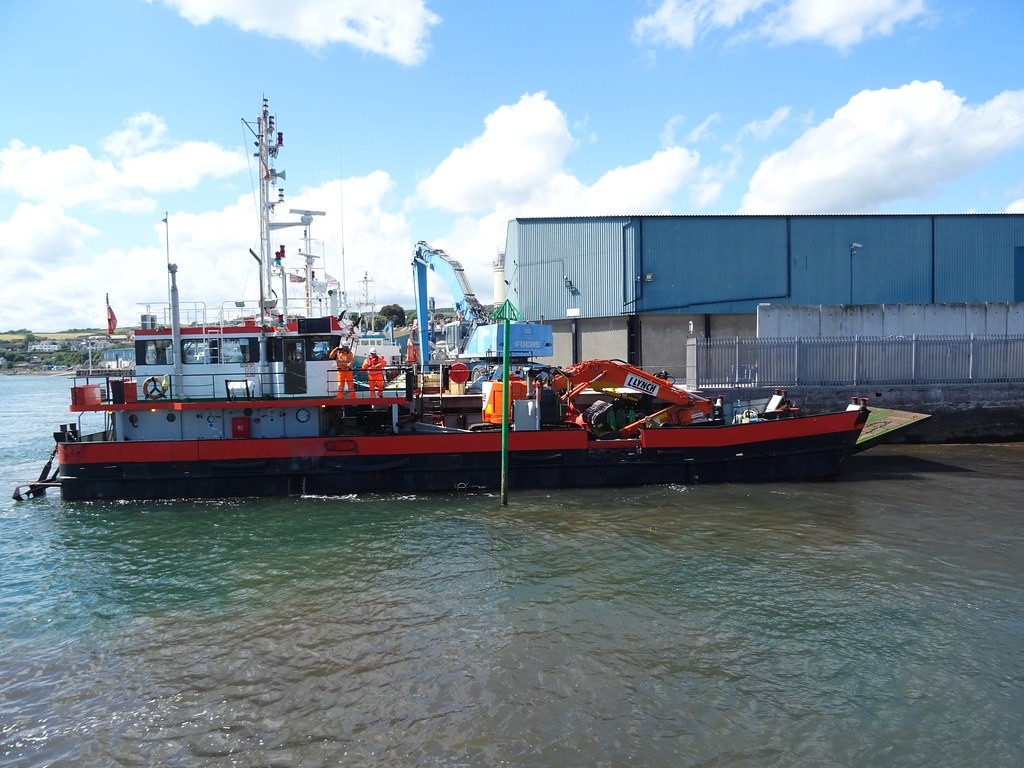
[359, 348, 387, 397]
[330, 340, 356, 398]
[311, 340, 326, 360]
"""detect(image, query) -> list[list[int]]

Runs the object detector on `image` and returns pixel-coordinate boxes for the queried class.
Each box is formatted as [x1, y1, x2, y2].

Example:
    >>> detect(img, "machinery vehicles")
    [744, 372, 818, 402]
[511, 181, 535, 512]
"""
[468, 352, 715, 441]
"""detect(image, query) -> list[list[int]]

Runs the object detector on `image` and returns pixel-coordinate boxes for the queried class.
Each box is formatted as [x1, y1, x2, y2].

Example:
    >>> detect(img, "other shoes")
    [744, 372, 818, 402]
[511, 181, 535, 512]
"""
[377, 393, 383, 398]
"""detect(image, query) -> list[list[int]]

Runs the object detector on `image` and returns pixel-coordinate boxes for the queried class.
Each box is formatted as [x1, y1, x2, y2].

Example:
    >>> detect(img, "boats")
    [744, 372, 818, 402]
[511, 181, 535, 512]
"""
[10, 83, 933, 505]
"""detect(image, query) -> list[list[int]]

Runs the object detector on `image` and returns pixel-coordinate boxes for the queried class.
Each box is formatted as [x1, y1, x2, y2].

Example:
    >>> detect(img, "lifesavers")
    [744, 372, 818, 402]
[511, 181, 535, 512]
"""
[143, 377, 166, 399]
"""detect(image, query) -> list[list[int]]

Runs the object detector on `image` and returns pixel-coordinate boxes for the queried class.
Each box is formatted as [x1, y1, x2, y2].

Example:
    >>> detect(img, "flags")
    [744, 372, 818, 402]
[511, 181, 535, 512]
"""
[106, 300, 117, 335]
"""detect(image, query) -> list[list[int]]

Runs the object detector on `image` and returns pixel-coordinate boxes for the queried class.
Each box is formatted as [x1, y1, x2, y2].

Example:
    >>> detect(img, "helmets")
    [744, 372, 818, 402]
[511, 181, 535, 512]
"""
[342, 341, 350, 346]
[369, 348, 376, 353]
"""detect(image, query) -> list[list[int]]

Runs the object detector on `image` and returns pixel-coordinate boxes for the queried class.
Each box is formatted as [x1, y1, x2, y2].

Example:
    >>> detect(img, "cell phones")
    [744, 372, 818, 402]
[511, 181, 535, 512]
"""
[337, 348, 343, 351]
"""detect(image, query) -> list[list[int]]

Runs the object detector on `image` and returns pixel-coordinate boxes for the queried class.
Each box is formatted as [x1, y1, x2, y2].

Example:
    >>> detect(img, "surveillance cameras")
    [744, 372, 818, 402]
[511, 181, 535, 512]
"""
[852, 243, 863, 248]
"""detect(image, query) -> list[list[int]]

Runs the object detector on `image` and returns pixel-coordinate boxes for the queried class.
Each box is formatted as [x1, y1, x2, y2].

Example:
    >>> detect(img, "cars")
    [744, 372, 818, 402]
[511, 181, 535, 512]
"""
[41, 364, 69, 372]
